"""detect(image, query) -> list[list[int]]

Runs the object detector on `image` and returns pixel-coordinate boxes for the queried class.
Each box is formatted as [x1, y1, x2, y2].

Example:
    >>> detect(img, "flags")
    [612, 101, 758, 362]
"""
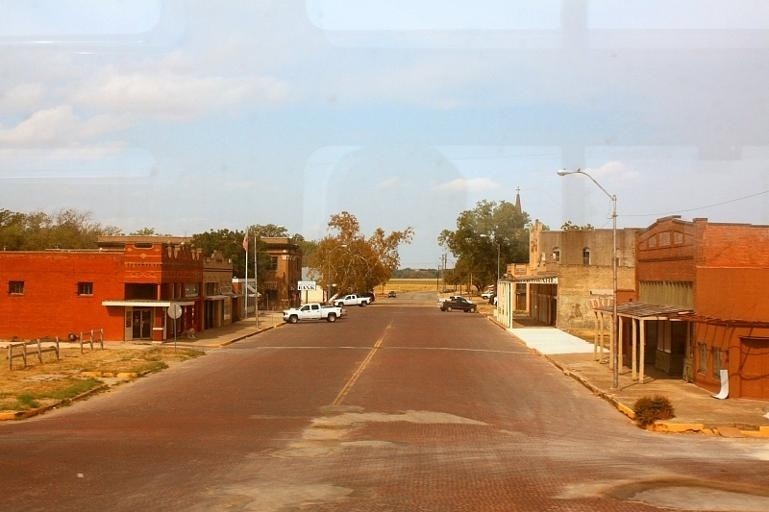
[243, 229, 248, 250]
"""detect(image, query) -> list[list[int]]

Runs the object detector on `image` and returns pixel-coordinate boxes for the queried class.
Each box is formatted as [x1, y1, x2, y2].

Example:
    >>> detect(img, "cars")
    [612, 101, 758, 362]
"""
[388, 291, 396, 298]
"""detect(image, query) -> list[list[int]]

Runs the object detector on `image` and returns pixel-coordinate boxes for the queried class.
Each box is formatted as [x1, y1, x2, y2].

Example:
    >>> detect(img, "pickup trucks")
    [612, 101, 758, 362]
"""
[333, 294, 371, 308]
[283, 303, 342, 324]
[438, 295, 477, 313]
[481, 291, 493, 299]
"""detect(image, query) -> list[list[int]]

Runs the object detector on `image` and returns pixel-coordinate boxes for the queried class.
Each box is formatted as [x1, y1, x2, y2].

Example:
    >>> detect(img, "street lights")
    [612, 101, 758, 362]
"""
[556, 168, 620, 388]
[480, 234, 501, 281]
[328, 244, 347, 297]
